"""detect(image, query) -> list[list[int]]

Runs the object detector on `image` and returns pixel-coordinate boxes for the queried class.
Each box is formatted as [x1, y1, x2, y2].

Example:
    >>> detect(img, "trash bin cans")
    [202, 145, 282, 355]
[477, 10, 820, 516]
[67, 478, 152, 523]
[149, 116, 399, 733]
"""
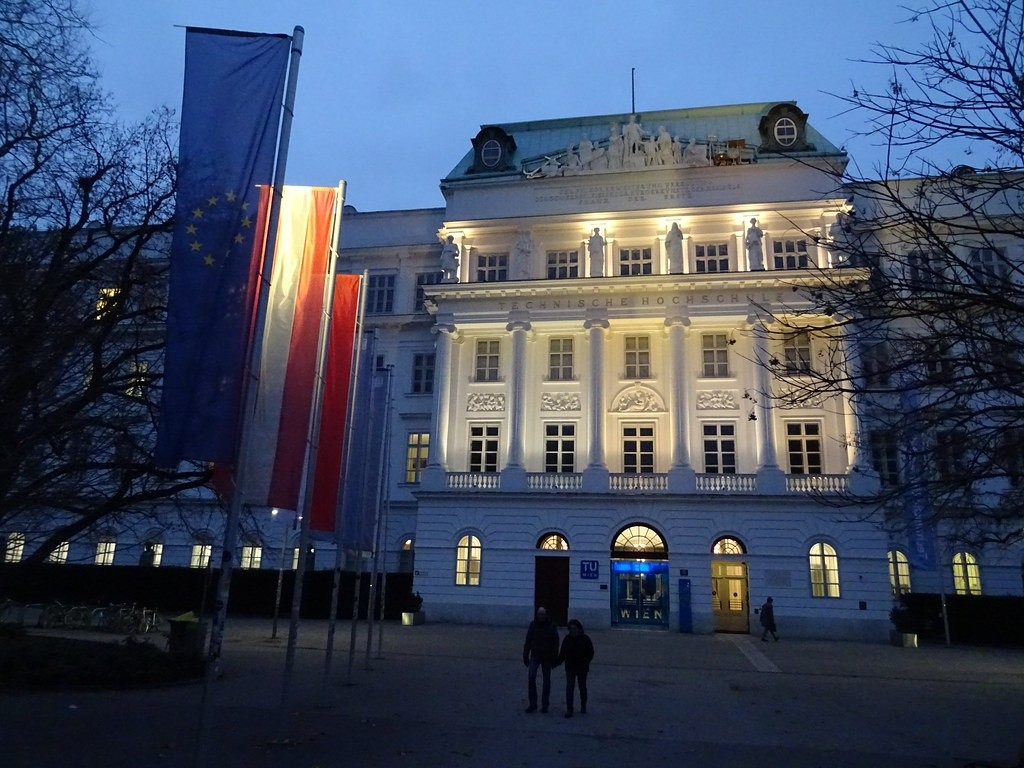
[167, 612, 208, 679]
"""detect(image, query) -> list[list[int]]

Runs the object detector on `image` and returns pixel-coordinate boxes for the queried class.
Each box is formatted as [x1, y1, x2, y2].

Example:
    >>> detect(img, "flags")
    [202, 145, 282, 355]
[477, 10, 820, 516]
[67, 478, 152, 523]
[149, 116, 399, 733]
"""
[150, 25, 293, 465]
[311, 329, 393, 555]
[303, 274, 363, 532]
[221, 183, 340, 511]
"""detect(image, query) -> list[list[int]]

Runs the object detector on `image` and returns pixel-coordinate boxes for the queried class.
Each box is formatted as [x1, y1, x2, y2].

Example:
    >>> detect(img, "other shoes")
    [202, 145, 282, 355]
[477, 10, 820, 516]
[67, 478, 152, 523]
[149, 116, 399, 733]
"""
[525, 706, 537, 713]
[539, 708, 548, 714]
[564, 710, 573, 717]
[580, 711, 588, 718]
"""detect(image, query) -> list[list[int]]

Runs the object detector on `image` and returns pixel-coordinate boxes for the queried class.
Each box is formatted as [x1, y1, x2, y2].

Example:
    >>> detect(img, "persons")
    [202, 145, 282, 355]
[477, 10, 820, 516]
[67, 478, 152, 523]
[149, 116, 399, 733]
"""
[522, 604, 562, 714]
[588, 227, 607, 275]
[439, 235, 460, 279]
[515, 231, 535, 277]
[665, 222, 684, 273]
[539, 114, 703, 173]
[759, 597, 781, 642]
[828, 213, 848, 263]
[551, 618, 595, 720]
[745, 218, 764, 269]
